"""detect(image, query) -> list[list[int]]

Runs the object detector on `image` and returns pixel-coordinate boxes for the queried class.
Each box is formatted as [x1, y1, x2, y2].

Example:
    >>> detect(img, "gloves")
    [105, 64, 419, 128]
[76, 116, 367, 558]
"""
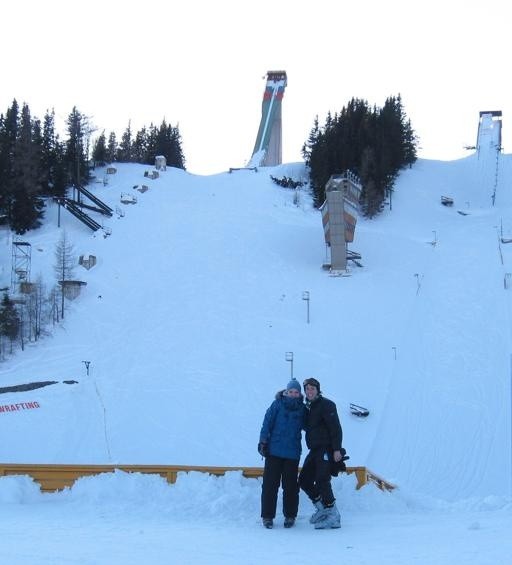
[257, 442, 269, 456]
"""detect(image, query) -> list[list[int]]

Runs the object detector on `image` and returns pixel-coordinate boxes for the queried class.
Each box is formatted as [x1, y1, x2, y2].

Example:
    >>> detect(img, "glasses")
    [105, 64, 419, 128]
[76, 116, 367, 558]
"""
[303, 379, 318, 386]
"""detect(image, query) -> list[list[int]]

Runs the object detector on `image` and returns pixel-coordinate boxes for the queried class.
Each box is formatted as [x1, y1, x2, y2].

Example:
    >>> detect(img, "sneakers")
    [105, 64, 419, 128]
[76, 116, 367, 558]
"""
[262, 517, 273, 527]
[284, 517, 295, 528]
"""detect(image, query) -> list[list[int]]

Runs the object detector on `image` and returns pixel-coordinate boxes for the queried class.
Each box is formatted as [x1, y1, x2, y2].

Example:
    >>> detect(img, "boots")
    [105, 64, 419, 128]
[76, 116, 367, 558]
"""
[309, 495, 328, 524]
[314, 500, 342, 529]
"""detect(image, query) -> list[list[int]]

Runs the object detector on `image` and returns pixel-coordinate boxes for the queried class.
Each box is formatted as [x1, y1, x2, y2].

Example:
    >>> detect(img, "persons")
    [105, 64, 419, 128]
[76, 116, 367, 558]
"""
[257, 376, 309, 528]
[298, 378, 343, 530]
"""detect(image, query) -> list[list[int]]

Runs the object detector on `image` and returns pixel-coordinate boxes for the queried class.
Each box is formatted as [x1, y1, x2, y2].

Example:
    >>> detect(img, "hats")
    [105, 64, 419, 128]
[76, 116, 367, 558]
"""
[286, 377, 301, 393]
